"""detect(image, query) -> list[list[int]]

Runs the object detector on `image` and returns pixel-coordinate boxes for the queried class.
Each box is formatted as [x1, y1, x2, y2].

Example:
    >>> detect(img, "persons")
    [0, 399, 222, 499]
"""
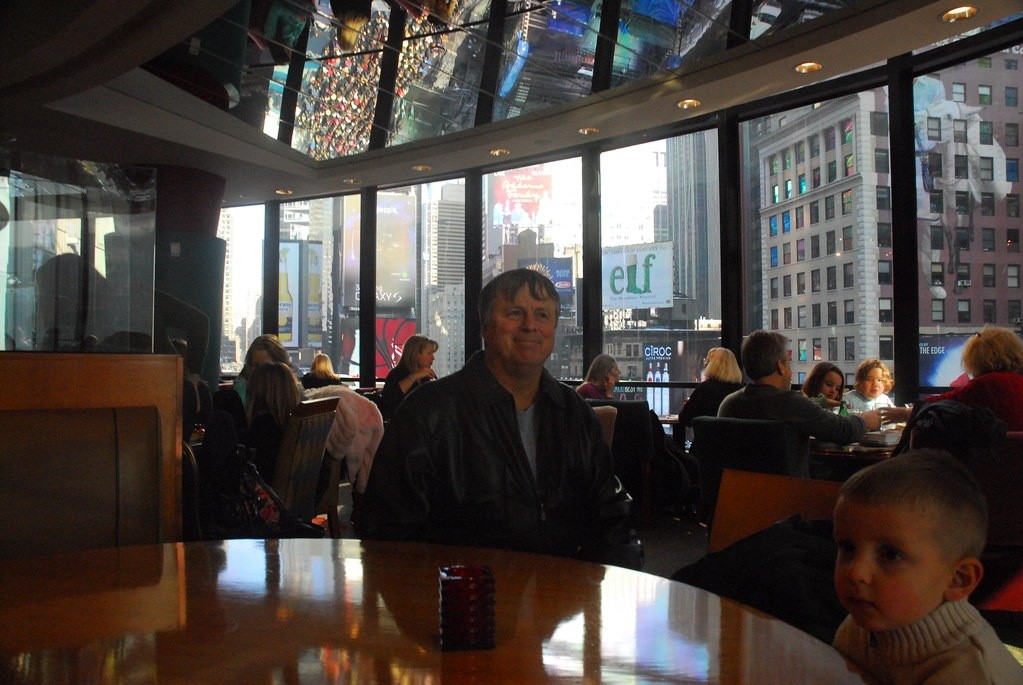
[679, 347, 742, 459]
[172, 338, 187, 356]
[802, 361, 848, 416]
[575, 354, 621, 399]
[234, 334, 343, 506]
[842, 356, 896, 414]
[4, 324, 99, 350]
[717, 329, 881, 480]
[361, 268, 645, 571]
[382, 334, 438, 415]
[877, 323, 1023, 431]
[830, 447, 1023, 685]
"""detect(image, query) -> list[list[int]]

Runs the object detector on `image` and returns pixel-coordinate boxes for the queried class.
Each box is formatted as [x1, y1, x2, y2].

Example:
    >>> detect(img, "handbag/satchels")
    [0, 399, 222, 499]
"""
[203, 445, 294, 536]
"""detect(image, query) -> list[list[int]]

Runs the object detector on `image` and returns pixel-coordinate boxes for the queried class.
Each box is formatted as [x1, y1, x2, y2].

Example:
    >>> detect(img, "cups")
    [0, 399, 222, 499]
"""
[875, 403, 889, 428]
[859, 406, 873, 414]
[437, 565, 496, 652]
[905, 403, 915, 407]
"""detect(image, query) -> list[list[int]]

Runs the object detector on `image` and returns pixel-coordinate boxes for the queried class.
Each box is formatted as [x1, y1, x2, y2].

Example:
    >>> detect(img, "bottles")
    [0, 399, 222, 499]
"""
[647, 362, 671, 427]
[838, 401, 848, 420]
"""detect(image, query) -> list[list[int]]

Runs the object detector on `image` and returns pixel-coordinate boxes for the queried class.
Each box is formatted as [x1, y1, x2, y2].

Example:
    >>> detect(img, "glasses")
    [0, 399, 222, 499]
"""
[702, 358, 708, 365]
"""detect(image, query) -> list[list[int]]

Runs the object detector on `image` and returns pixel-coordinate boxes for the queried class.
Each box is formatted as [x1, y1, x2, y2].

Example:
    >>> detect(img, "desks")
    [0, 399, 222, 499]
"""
[657, 414, 685, 452]
[0, 538, 878, 685]
[807, 439, 897, 458]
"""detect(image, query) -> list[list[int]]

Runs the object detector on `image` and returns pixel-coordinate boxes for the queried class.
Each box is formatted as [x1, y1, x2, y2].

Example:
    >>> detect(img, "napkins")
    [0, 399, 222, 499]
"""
[868, 431, 898, 442]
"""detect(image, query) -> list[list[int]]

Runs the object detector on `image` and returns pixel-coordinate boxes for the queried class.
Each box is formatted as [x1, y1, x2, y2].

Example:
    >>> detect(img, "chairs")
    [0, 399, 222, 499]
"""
[182, 387, 650, 613]
[708, 468, 844, 553]
[911, 428, 1023, 545]
[693, 414, 785, 514]
